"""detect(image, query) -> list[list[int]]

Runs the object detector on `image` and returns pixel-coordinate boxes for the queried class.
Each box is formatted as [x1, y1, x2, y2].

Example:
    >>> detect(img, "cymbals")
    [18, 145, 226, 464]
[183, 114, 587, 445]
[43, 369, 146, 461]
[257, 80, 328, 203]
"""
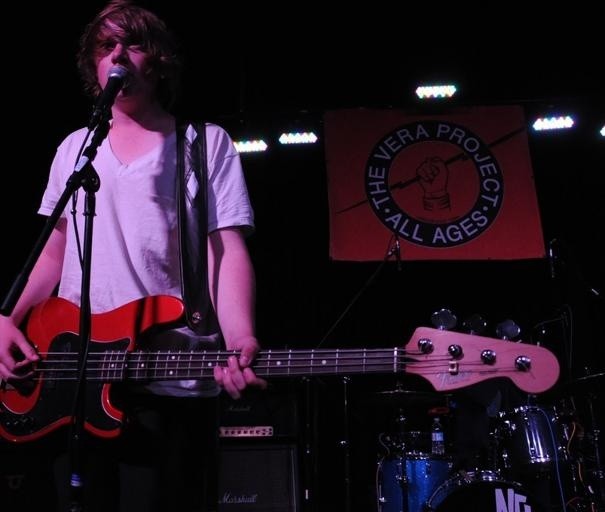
[373, 388, 428, 395]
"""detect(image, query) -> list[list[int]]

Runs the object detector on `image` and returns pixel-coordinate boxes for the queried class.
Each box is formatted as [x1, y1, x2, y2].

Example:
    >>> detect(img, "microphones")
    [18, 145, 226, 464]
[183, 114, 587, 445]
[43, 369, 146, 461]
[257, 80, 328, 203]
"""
[395, 240, 403, 271]
[86, 65, 129, 130]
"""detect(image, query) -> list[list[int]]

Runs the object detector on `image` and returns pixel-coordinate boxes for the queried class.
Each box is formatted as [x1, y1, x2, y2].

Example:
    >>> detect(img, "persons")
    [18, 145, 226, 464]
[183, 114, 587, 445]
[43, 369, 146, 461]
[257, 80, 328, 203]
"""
[0, 0, 271, 512]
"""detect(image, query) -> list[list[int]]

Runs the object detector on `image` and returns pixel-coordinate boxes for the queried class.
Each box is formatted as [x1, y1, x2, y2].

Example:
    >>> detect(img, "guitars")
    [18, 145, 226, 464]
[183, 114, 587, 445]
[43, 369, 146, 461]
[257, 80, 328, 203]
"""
[0, 295, 560, 443]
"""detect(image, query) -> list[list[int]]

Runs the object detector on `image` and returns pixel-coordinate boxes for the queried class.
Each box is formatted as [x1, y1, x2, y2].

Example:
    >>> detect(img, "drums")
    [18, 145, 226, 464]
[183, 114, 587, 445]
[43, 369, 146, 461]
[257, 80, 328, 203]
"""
[424, 467, 542, 512]
[377, 452, 453, 511]
[488, 405, 568, 482]
